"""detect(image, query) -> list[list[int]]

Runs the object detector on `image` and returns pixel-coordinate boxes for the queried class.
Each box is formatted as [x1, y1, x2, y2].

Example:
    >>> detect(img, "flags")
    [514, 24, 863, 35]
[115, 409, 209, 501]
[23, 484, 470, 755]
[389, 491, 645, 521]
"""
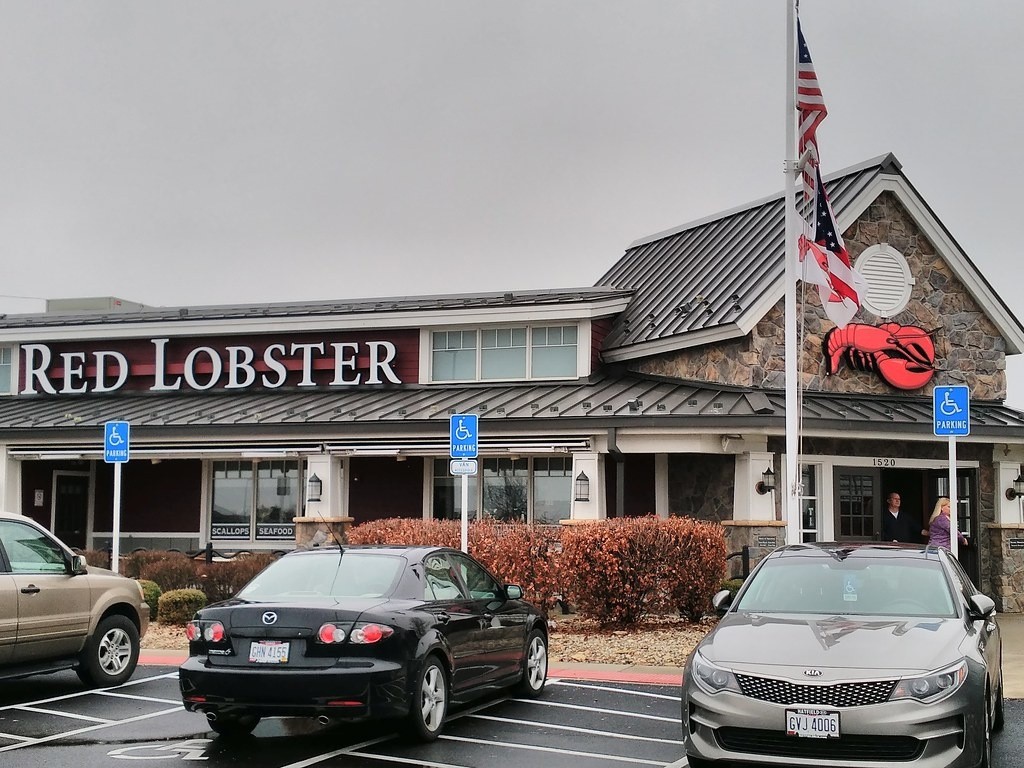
[795, 10, 870, 330]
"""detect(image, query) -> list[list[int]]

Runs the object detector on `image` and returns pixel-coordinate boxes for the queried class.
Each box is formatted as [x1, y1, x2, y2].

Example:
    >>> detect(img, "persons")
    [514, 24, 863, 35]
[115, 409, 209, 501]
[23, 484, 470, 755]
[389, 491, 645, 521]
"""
[883, 491, 929, 543]
[929, 497, 968, 553]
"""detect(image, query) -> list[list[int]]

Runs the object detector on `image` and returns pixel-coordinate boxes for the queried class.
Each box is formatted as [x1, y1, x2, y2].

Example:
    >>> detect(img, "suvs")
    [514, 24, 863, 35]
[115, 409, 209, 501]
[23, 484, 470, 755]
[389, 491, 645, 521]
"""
[0, 509, 153, 689]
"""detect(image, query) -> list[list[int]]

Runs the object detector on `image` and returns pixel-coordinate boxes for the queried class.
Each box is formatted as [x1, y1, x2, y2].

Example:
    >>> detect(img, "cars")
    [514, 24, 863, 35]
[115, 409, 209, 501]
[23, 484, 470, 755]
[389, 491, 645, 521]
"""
[177, 544, 551, 741]
[678, 540, 1007, 768]
